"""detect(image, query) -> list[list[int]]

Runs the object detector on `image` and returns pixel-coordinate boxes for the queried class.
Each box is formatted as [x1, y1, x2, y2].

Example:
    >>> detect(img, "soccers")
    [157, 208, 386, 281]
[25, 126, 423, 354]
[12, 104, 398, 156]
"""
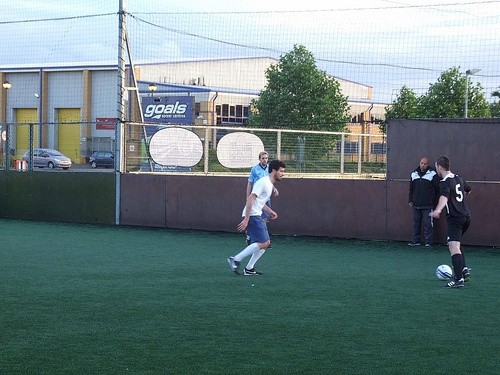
[436, 264, 452, 280]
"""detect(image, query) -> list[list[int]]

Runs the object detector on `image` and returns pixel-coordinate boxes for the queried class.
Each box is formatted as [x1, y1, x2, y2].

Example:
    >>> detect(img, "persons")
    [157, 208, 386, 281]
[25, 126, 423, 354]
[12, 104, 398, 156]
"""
[407, 157, 441, 247]
[429, 155, 472, 287]
[227, 160, 287, 276]
[245, 152, 280, 249]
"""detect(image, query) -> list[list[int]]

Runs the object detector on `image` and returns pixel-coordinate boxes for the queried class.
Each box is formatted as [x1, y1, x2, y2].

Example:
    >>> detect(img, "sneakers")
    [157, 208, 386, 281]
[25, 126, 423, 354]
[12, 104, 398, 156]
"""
[246, 229, 251, 245]
[425, 242, 433, 246]
[444, 277, 464, 287]
[243, 267, 263, 275]
[408, 240, 421, 245]
[227, 256, 242, 274]
[462, 266, 470, 282]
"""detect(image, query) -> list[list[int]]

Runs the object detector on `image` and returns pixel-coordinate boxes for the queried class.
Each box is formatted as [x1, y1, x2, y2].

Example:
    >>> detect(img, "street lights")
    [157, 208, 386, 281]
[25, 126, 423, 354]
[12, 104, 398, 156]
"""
[148, 83, 158, 97]
[2, 81, 11, 122]
[464, 68, 483, 119]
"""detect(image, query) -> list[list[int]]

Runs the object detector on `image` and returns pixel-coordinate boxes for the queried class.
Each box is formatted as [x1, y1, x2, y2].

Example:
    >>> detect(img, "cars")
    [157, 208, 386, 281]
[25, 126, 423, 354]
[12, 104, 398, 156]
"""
[89, 150, 115, 169]
[22, 149, 72, 170]
